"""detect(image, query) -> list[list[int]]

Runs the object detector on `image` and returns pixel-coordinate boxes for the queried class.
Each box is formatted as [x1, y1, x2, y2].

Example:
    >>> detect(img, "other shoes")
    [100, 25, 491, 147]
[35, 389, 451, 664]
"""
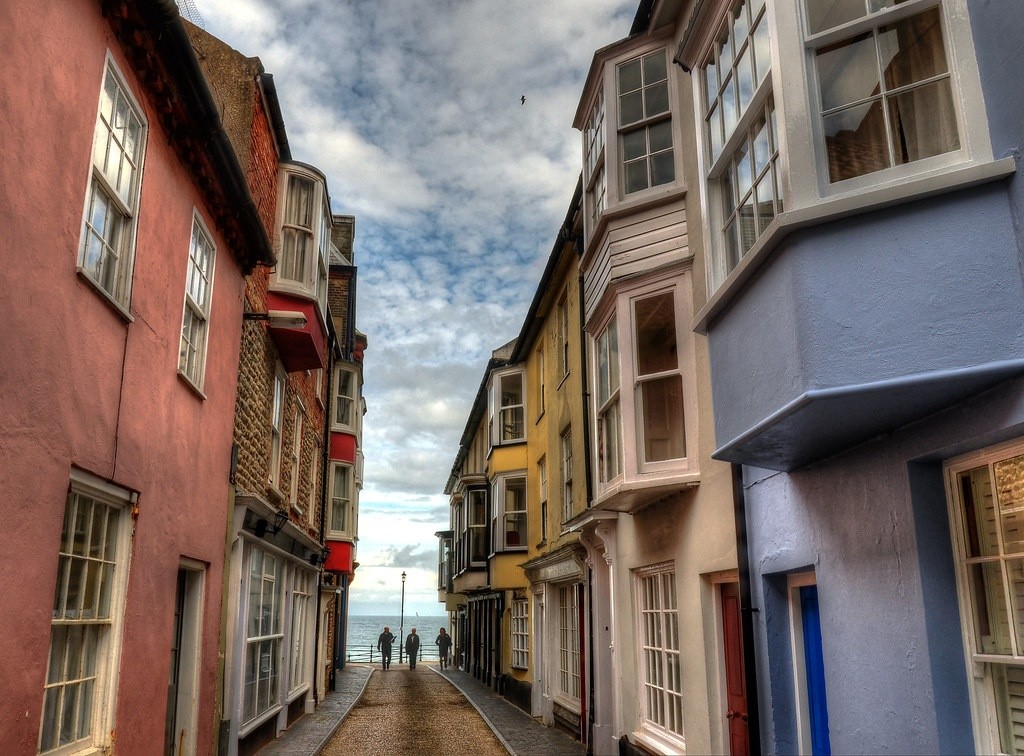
[383, 666, 386, 671]
[410, 665, 412, 670]
[445, 663, 447, 668]
[387, 663, 389, 669]
[414, 665, 416, 670]
[441, 666, 443, 669]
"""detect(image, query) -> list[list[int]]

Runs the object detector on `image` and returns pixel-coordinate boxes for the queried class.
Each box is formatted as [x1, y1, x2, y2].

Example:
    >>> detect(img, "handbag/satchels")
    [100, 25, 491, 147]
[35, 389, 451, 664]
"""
[448, 641, 452, 646]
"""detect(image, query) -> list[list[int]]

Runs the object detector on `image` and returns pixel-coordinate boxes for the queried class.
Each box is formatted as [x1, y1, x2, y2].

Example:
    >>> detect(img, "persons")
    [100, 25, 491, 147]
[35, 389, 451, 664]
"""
[435, 627, 452, 670]
[405, 628, 420, 671]
[377, 627, 395, 671]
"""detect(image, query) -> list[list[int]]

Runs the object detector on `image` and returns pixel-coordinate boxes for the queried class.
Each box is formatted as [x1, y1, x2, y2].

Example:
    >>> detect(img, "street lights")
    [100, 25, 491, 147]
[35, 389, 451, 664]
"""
[398, 570, 407, 663]
